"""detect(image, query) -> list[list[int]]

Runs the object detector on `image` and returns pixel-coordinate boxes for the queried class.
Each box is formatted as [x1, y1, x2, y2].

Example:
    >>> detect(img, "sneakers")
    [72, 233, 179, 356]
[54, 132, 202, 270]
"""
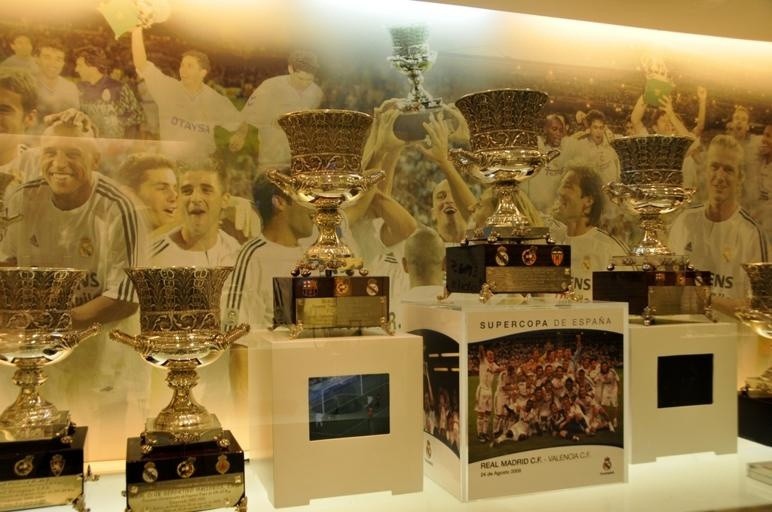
[478, 430, 501, 444]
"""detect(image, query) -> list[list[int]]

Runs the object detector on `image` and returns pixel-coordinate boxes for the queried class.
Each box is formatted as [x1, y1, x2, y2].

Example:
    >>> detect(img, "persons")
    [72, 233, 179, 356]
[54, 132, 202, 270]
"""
[423, 389, 459, 455]
[476, 334, 619, 447]
[0, 9, 771, 317]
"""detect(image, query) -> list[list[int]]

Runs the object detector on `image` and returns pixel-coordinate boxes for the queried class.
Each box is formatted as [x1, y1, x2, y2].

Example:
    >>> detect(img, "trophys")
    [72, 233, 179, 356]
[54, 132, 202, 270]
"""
[0, 267, 103, 446]
[737, 263, 772, 395]
[111, 268, 251, 455]
